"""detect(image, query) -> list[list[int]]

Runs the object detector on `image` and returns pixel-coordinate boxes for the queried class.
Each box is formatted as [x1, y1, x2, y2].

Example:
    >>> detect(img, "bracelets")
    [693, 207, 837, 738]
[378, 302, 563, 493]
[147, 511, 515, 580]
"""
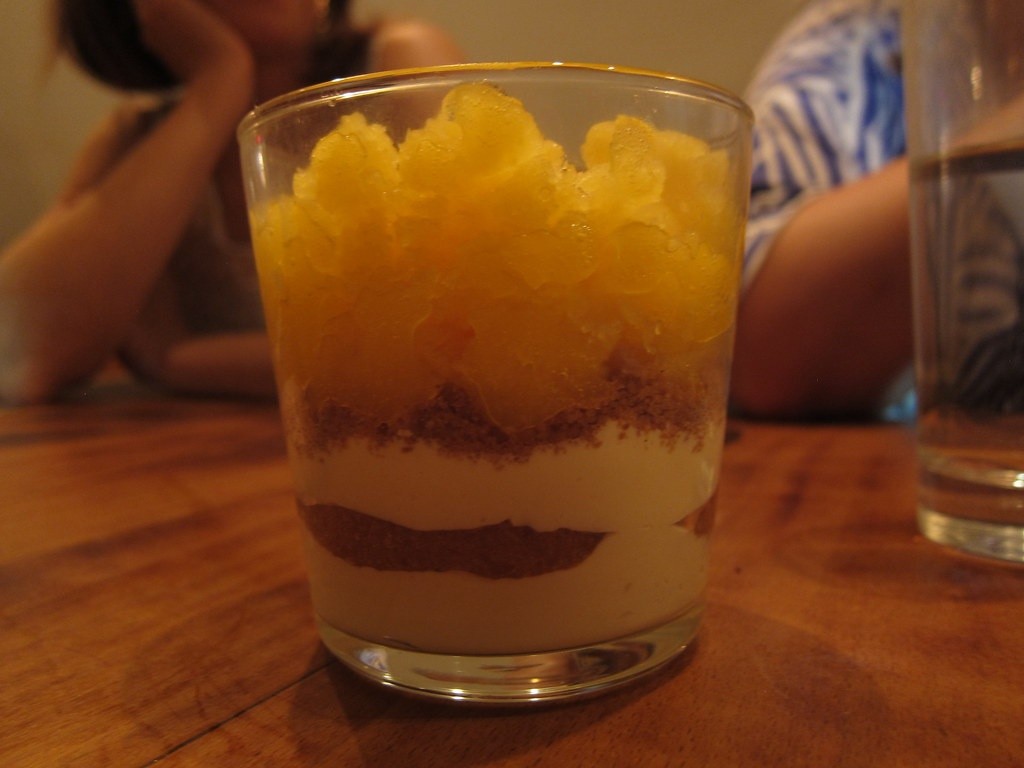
[116, 345, 173, 398]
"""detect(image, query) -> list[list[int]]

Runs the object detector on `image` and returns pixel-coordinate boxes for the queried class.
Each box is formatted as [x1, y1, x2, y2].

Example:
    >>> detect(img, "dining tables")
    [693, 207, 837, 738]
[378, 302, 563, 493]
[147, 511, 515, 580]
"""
[1, 397, 1024, 767]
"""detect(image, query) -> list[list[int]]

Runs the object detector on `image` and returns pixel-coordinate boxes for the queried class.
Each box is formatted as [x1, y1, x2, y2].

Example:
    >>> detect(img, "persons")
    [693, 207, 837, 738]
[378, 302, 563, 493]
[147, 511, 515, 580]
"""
[725, 0, 1024, 414]
[0, 0, 487, 403]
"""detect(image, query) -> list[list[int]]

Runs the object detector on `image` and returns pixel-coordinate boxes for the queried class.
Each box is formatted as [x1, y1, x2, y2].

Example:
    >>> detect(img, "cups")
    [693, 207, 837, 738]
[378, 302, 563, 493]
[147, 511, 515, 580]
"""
[236, 62, 756, 705]
[899, 1, 1023, 560]
